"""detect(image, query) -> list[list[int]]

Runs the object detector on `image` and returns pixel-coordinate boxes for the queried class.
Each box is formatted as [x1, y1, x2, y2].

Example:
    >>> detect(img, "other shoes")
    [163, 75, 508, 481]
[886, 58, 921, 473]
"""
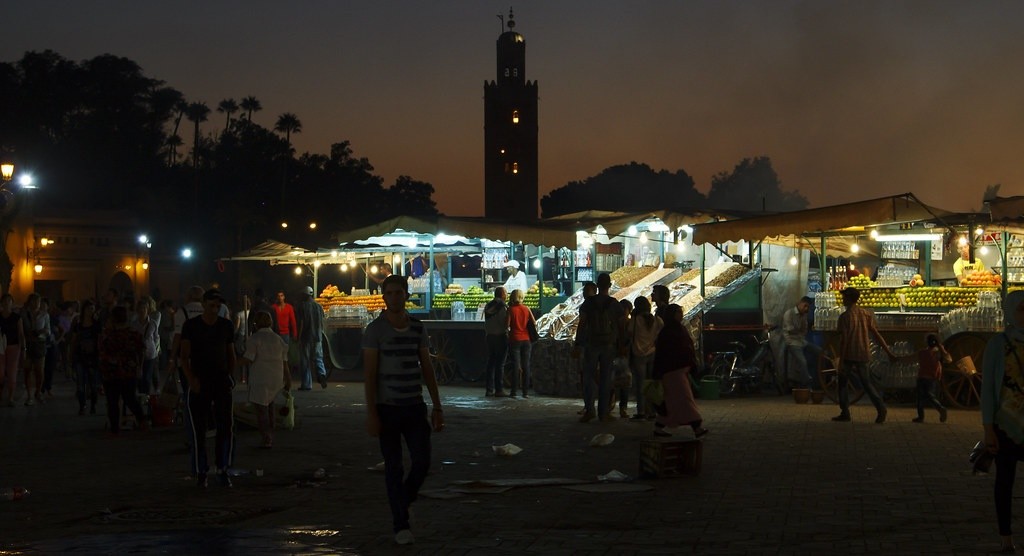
[298, 387, 311, 390]
[875, 409, 887, 424]
[36, 392, 44, 402]
[1000, 538, 1016, 556]
[523, 394, 528, 398]
[912, 415, 924, 422]
[608, 398, 616, 411]
[600, 415, 617, 422]
[653, 430, 672, 437]
[24, 399, 33, 406]
[139, 421, 145, 431]
[106, 431, 122, 436]
[832, 414, 850, 422]
[496, 390, 510, 397]
[631, 413, 656, 422]
[319, 375, 327, 389]
[939, 406, 947, 423]
[509, 392, 516, 397]
[695, 429, 708, 438]
[577, 407, 586, 414]
[8, 401, 16, 407]
[620, 406, 630, 418]
[580, 413, 595, 423]
[486, 390, 495, 396]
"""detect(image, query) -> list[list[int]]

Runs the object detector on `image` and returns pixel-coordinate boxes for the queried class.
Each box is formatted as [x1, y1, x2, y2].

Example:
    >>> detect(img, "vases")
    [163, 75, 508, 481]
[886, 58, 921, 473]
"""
[811, 391, 826, 403]
[793, 388, 811, 404]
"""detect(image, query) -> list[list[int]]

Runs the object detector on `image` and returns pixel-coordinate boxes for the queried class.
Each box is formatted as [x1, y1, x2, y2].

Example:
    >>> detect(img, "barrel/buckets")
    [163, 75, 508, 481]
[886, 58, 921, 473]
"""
[151, 405, 173, 425]
[956, 355, 977, 376]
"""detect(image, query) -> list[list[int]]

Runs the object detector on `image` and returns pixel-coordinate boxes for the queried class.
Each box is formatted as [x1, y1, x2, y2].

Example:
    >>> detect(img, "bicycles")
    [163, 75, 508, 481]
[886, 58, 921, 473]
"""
[712, 326, 788, 394]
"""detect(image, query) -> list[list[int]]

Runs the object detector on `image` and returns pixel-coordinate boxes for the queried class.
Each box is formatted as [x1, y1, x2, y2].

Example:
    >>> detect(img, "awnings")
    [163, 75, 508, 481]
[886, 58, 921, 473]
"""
[695, 193, 1024, 290]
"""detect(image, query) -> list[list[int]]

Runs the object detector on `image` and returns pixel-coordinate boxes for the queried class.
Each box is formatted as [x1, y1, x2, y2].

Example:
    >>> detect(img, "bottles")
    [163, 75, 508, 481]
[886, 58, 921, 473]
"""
[875, 313, 937, 330]
[881, 242, 942, 259]
[815, 307, 840, 330]
[977, 292, 1000, 306]
[327, 305, 382, 328]
[483, 249, 506, 269]
[378, 285, 381, 294]
[451, 301, 465, 320]
[937, 306, 1003, 338]
[829, 266, 847, 291]
[809, 275, 821, 291]
[351, 287, 370, 296]
[1000, 235, 1024, 282]
[578, 268, 592, 281]
[893, 341, 914, 354]
[867, 341, 889, 377]
[877, 267, 918, 286]
[407, 268, 441, 290]
[0, 487, 31, 501]
[884, 363, 920, 388]
[815, 292, 835, 307]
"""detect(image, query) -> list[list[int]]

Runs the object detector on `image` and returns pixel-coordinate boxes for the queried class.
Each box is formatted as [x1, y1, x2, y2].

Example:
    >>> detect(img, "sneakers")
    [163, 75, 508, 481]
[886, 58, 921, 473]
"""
[196, 473, 207, 488]
[214, 466, 226, 480]
[396, 530, 415, 545]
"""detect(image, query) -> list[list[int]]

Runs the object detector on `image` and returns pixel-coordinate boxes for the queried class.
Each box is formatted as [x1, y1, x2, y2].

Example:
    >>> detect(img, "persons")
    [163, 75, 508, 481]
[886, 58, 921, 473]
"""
[782, 296, 824, 390]
[892, 333, 952, 423]
[831, 287, 898, 424]
[969, 291, 1024, 556]
[0, 286, 328, 491]
[485, 288, 539, 397]
[574, 273, 709, 438]
[362, 276, 445, 544]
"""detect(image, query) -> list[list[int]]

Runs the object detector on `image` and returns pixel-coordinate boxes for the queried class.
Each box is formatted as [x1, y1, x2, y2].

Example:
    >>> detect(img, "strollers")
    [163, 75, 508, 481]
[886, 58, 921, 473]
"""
[103, 392, 154, 432]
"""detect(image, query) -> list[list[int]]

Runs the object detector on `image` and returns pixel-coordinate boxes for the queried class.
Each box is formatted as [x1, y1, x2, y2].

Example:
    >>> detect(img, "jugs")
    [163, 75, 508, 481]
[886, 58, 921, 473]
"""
[689, 375, 722, 400]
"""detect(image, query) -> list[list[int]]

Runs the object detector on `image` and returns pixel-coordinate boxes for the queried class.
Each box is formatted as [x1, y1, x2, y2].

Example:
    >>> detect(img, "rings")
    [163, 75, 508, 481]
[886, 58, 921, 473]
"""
[440, 423, 445, 426]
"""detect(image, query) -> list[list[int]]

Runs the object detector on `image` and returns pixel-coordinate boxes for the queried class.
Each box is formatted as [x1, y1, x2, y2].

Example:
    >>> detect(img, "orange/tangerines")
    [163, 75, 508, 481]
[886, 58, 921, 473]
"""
[910, 274, 924, 287]
[961, 270, 1001, 286]
[314, 295, 418, 313]
[320, 285, 346, 297]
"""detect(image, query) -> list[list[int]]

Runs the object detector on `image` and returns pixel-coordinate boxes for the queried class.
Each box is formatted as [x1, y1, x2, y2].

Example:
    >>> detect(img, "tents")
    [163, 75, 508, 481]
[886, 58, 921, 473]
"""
[219, 207, 750, 326]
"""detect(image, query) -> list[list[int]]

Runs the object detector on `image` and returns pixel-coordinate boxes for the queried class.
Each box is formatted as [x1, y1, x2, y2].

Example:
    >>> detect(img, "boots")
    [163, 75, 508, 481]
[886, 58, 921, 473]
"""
[89, 398, 96, 415]
[78, 401, 86, 415]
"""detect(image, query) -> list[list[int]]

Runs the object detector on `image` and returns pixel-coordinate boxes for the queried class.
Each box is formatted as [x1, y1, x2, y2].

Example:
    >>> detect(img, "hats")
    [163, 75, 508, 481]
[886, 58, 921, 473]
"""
[204, 289, 225, 305]
[840, 288, 859, 299]
[300, 286, 313, 296]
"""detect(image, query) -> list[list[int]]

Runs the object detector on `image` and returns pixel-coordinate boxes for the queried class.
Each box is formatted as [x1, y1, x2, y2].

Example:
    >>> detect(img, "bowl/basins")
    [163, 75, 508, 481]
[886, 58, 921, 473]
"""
[792, 389, 809, 403]
[811, 391, 824, 403]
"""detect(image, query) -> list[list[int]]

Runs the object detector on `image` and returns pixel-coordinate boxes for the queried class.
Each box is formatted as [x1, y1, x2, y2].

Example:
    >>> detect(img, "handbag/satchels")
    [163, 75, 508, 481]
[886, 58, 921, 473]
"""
[272, 390, 294, 431]
[526, 320, 539, 343]
[32, 341, 47, 358]
[120, 360, 143, 384]
[629, 344, 633, 368]
[158, 367, 184, 410]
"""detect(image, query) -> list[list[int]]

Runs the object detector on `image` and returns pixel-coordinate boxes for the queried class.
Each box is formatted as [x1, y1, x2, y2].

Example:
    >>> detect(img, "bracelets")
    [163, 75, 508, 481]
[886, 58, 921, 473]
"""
[433, 408, 444, 413]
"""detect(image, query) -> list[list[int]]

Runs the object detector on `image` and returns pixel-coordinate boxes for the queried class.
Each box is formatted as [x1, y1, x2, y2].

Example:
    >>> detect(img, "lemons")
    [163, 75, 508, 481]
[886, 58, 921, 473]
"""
[845, 274, 875, 288]
[528, 281, 560, 297]
[830, 287, 992, 308]
[433, 294, 539, 309]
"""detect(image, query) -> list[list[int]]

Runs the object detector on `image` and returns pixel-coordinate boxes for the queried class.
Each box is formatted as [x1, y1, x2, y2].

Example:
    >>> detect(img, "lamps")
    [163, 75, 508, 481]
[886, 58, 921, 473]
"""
[875, 228, 945, 242]
[142, 259, 149, 272]
[33, 255, 43, 275]
[26, 233, 49, 263]
[0, 162, 17, 192]
